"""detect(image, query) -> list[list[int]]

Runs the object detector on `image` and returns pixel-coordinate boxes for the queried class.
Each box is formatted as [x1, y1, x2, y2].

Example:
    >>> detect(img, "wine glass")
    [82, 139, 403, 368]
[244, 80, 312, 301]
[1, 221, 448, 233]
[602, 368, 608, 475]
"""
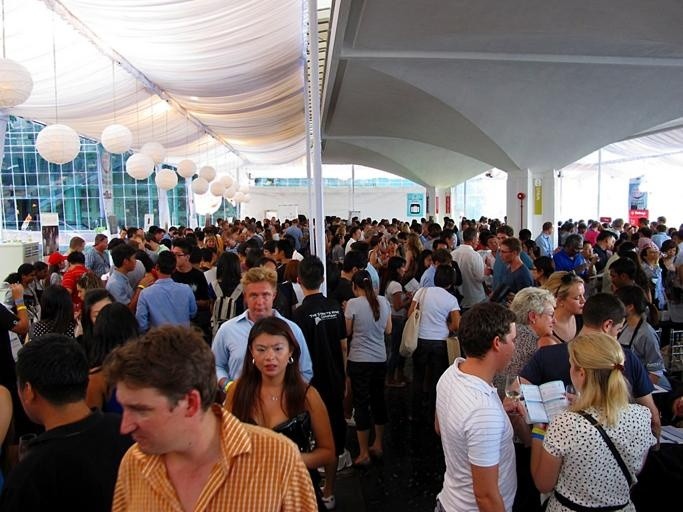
[0, 281, 19, 290]
[504, 373, 521, 418]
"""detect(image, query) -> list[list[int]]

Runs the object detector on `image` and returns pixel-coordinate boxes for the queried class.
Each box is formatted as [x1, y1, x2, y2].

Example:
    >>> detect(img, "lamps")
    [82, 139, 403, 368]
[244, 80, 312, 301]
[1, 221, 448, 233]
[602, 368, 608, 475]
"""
[0, 0, 134, 179]
[135, 71, 248, 202]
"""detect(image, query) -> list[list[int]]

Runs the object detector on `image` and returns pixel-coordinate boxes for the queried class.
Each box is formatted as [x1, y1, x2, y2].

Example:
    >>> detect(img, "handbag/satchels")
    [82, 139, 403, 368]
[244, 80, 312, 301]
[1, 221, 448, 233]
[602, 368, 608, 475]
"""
[398, 308, 422, 358]
[271, 410, 317, 453]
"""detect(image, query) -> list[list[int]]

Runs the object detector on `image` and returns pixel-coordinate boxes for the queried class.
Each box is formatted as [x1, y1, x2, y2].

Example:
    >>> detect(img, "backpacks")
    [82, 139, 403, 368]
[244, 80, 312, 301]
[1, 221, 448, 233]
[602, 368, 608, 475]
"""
[208, 279, 243, 336]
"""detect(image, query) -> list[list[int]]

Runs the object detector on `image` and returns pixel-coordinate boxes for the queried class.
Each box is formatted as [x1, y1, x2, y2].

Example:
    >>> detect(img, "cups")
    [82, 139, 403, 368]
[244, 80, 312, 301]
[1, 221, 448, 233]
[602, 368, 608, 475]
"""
[564, 386, 579, 408]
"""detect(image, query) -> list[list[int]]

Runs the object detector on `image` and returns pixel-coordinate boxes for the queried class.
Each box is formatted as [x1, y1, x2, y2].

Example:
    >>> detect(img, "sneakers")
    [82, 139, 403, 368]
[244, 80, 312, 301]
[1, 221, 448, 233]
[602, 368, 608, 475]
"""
[319, 486, 336, 511]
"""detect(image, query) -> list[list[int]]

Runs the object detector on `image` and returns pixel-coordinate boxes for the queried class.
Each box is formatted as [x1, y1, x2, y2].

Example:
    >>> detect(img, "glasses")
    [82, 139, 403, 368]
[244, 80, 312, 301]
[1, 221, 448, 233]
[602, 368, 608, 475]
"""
[554, 269, 576, 299]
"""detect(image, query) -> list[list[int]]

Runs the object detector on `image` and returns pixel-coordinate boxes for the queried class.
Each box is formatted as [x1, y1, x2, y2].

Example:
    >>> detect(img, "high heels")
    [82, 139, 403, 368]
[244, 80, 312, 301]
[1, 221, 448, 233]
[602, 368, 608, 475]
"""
[369, 445, 384, 459]
[354, 452, 371, 465]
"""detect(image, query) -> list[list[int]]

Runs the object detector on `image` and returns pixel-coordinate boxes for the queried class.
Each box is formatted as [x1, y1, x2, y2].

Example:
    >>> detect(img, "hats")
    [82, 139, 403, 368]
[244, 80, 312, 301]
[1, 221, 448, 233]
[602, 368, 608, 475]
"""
[48, 252, 69, 265]
[637, 237, 653, 250]
